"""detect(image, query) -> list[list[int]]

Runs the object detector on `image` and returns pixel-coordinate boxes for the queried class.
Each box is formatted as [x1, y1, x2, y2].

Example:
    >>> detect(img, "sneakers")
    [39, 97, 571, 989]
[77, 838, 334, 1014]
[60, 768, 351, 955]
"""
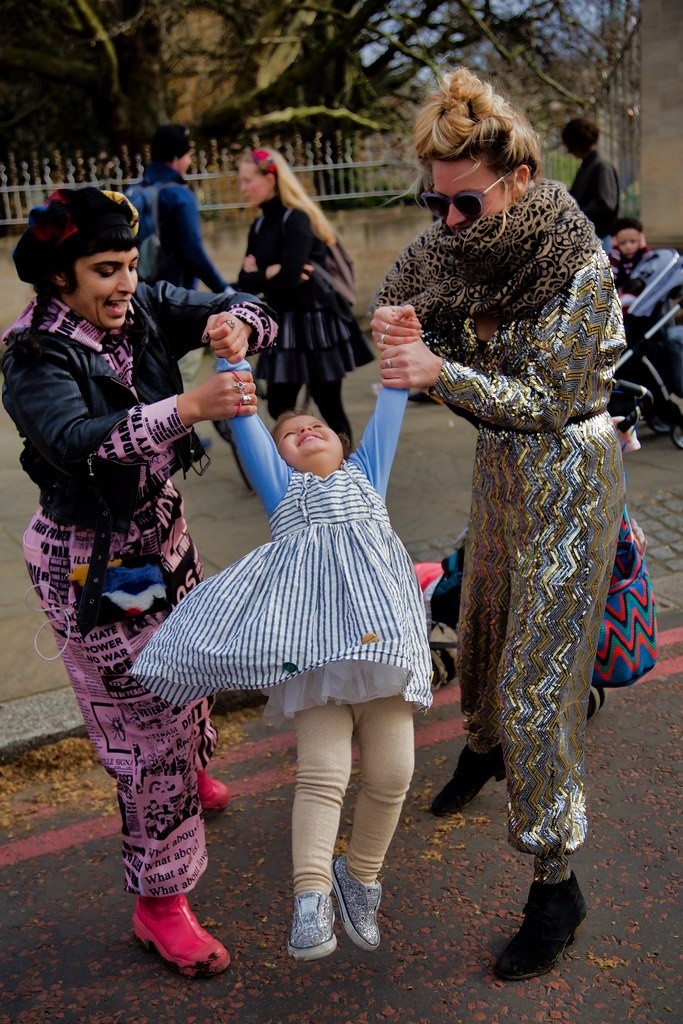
[331, 856, 383, 951]
[286, 891, 338, 962]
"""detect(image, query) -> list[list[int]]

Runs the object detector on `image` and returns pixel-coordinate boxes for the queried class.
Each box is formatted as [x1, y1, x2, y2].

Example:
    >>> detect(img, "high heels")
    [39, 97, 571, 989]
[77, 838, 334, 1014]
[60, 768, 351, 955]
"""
[194, 768, 231, 813]
[495, 869, 587, 980]
[134, 891, 231, 979]
[430, 741, 506, 817]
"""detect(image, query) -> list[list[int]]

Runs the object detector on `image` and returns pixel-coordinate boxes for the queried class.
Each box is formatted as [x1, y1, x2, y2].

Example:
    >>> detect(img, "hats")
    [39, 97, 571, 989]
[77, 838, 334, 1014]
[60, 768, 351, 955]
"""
[611, 218, 642, 236]
[151, 125, 195, 163]
[13, 187, 142, 284]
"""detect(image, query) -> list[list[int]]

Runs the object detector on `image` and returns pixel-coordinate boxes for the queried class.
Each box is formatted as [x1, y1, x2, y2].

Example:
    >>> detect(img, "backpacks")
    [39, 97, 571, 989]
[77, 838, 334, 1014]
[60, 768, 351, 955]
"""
[124, 181, 189, 284]
[256, 207, 356, 306]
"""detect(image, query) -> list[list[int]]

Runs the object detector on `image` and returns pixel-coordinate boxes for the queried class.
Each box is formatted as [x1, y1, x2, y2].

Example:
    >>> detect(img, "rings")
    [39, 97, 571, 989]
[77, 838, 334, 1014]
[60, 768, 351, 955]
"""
[225, 319, 235, 328]
[381, 334, 385, 344]
[233, 382, 246, 389]
[242, 396, 252, 404]
[386, 324, 390, 335]
[386, 359, 392, 369]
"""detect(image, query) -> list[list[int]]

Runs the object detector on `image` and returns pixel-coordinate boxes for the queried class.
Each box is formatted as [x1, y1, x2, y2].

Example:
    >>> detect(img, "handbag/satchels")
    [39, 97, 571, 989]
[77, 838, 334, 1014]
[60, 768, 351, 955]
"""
[79, 554, 168, 627]
[590, 540, 658, 688]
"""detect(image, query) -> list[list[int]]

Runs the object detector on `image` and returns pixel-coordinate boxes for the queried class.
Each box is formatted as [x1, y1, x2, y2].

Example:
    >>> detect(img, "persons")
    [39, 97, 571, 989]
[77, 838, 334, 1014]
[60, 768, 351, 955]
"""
[560, 118, 652, 294]
[236, 146, 378, 451]
[0, 188, 279, 978]
[367, 66, 657, 981]
[125, 123, 231, 452]
[128, 311, 433, 963]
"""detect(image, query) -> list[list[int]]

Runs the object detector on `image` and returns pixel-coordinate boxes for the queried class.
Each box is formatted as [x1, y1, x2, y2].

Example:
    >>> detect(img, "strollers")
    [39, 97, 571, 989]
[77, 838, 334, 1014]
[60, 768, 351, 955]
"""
[605, 249, 683, 452]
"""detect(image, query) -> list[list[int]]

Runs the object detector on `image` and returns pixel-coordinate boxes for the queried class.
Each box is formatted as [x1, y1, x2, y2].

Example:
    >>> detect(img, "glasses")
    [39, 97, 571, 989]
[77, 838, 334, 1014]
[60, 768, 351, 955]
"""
[421, 163, 534, 218]
